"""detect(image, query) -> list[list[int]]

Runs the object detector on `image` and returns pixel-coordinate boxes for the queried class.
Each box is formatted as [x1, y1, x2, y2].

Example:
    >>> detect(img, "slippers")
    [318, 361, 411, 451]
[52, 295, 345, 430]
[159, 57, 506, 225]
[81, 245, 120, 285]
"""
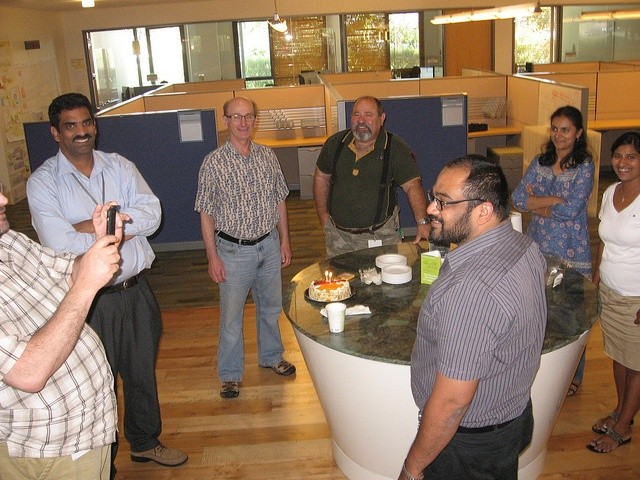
[592, 412, 634, 434]
[586, 429, 631, 454]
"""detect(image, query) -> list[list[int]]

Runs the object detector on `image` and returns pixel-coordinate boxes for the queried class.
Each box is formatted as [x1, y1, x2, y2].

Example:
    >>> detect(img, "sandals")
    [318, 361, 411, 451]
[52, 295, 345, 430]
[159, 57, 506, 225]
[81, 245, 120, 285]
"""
[567, 383, 579, 395]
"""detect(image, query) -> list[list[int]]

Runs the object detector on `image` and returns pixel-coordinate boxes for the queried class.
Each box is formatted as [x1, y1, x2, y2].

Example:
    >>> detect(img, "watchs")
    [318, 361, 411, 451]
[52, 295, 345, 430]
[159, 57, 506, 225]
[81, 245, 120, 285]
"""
[401, 458, 426, 479]
[416, 217, 432, 226]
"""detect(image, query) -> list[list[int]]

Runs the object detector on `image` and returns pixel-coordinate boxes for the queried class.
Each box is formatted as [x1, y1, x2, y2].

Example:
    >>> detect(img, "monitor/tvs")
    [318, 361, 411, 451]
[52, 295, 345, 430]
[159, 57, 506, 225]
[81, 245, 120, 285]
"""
[419, 66, 436, 81]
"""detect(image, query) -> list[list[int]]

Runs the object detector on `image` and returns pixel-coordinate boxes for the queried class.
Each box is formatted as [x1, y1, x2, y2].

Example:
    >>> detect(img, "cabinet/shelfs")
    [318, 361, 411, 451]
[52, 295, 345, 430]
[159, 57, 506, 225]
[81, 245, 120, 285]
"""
[498, 152, 523, 191]
[296, 146, 325, 201]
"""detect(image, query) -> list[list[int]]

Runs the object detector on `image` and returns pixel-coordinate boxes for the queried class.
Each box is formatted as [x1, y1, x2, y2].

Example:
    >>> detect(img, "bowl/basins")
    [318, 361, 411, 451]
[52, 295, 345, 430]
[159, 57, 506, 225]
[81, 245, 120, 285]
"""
[376, 256, 408, 268]
[384, 267, 411, 284]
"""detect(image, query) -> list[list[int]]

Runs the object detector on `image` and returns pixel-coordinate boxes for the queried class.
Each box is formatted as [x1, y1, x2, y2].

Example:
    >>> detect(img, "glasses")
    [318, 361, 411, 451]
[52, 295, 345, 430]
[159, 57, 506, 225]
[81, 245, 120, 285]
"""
[225, 113, 255, 121]
[427, 193, 487, 211]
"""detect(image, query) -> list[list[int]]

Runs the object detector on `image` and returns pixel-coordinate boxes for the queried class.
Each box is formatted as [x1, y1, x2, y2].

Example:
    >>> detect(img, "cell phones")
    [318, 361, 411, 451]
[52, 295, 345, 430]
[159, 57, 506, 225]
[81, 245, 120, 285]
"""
[107, 206, 119, 239]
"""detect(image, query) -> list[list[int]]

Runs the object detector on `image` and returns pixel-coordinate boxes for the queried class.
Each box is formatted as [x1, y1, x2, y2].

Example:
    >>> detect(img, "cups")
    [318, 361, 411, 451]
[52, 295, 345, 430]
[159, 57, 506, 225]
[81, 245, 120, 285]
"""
[325, 303, 349, 334]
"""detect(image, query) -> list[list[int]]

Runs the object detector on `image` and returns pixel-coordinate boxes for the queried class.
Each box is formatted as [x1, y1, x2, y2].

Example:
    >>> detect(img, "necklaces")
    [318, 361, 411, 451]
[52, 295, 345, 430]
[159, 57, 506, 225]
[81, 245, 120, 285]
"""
[621, 181, 640, 203]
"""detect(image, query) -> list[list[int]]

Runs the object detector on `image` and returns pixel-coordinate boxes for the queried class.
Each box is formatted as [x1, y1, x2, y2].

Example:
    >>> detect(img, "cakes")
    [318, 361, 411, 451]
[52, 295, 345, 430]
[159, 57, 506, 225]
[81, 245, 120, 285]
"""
[309, 280, 351, 302]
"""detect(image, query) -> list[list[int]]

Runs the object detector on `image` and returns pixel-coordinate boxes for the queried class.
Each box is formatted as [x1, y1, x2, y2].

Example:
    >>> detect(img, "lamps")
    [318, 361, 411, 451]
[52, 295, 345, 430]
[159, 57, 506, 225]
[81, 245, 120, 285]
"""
[267, 0, 288, 34]
[430, 1, 542, 25]
[580, 4, 640, 21]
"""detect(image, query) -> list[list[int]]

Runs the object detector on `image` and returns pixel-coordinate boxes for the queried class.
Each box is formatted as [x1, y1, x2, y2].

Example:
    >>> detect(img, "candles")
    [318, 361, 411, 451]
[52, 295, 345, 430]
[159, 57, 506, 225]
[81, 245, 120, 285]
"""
[326, 271, 333, 283]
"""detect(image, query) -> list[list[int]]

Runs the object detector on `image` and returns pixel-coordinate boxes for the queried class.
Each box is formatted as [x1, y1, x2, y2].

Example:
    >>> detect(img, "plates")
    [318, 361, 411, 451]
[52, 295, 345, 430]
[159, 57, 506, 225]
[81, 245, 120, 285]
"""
[303, 283, 355, 300]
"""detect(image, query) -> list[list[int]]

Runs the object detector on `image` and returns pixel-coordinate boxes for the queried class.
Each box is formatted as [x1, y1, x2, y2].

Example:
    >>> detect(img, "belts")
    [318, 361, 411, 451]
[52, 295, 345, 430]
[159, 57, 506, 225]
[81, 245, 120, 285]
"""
[215, 230, 270, 246]
[329, 212, 393, 234]
[99, 275, 138, 296]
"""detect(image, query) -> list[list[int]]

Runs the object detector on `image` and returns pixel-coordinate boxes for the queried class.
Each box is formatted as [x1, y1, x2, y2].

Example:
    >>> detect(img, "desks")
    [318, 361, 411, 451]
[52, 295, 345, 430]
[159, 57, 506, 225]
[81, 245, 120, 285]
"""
[420, 77, 522, 150]
[281, 240, 603, 480]
[523, 71, 640, 218]
[216, 84, 326, 203]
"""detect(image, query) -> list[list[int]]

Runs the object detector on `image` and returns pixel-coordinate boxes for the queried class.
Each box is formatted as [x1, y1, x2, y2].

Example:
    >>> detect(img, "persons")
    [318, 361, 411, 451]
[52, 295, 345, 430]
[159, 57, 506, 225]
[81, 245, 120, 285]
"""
[511, 106, 595, 397]
[0, 192, 131, 480]
[25, 92, 188, 480]
[313, 96, 432, 261]
[584, 131, 640, 454]
[194, 96, 296, 399]
[397, 154, 549, 480]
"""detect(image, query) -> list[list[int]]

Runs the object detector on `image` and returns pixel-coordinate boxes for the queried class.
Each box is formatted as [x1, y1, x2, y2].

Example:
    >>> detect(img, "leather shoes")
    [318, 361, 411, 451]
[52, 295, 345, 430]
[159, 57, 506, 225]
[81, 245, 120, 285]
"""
[130, 444, 188, 467]
[220, 381, 240, 398]
[257, 360, 296, 376]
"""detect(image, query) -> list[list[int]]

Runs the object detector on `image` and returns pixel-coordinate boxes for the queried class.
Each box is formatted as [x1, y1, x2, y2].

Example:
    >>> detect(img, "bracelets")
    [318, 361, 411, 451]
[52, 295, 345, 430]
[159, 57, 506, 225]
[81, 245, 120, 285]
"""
[545, 206, 548, 218]
